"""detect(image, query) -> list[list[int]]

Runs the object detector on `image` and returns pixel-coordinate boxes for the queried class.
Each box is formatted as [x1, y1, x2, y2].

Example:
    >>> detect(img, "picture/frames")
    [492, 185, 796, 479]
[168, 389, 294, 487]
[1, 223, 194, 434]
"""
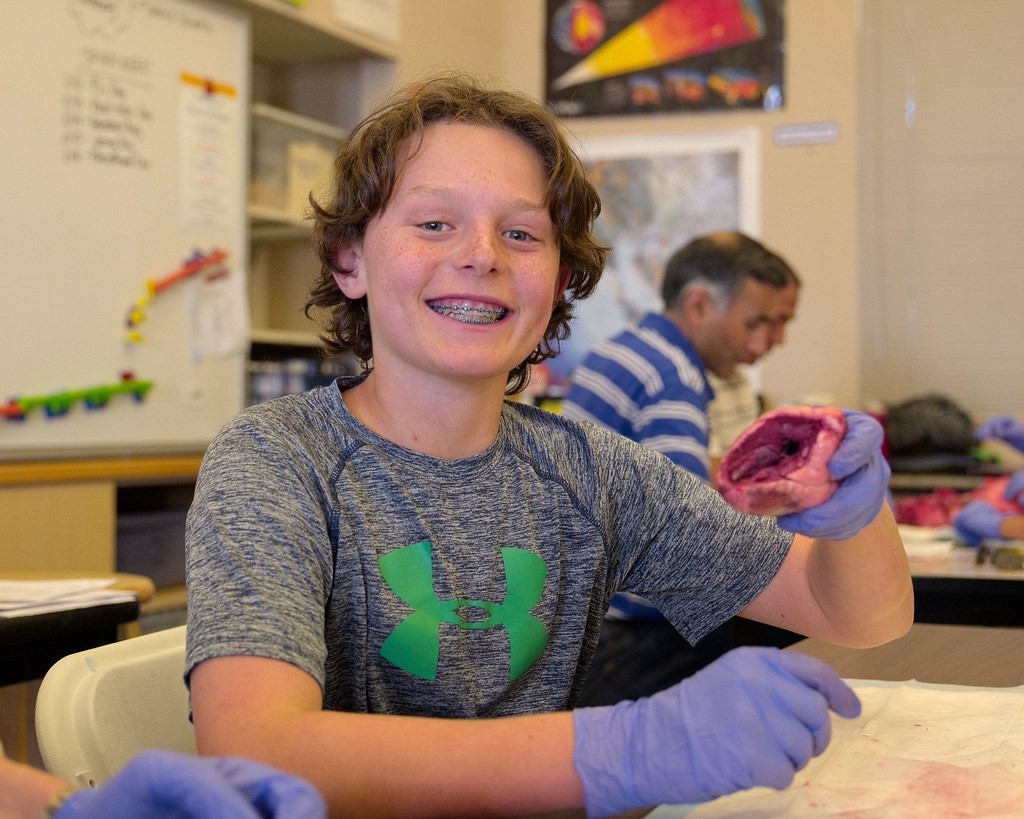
[517, 130, 761, 418]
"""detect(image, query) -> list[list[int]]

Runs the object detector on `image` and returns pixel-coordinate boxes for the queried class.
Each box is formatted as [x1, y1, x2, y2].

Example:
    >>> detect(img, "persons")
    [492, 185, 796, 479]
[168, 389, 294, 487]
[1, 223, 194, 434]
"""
[185, 71, 915, 817]
[758, 251, 802, 353]
[1, 749, 323, 819]
[565, 232, 783, 491]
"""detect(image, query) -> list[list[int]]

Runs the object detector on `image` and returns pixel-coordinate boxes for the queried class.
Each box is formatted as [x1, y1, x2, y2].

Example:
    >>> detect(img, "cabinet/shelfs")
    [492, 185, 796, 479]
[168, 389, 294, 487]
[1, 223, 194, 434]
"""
[0, 452, 207, 771]
[245, 0, 402, 415]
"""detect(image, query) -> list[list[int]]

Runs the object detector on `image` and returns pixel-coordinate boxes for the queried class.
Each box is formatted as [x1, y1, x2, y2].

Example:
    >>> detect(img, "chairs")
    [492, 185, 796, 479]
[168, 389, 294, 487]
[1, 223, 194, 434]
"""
[35, 624, 197, 798]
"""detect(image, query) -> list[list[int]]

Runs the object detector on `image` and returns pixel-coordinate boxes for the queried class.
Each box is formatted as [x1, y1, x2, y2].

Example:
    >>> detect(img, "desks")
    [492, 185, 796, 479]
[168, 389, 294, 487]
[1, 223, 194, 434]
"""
[1, 571, 155, 687]
[887, 518, 1024, 580]
[602, 623, 1024, 819]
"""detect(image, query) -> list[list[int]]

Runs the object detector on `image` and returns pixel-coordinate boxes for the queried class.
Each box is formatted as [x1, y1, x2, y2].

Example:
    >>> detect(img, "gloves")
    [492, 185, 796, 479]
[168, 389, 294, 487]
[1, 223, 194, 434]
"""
[975, 415, 1024, 455]
[573, 645, 862, 818]
[954, 499, 1014, 546]
[52, 747, 326, 819]
[780, 412, 895, 538]
[1005, 472, 1024, 503]
